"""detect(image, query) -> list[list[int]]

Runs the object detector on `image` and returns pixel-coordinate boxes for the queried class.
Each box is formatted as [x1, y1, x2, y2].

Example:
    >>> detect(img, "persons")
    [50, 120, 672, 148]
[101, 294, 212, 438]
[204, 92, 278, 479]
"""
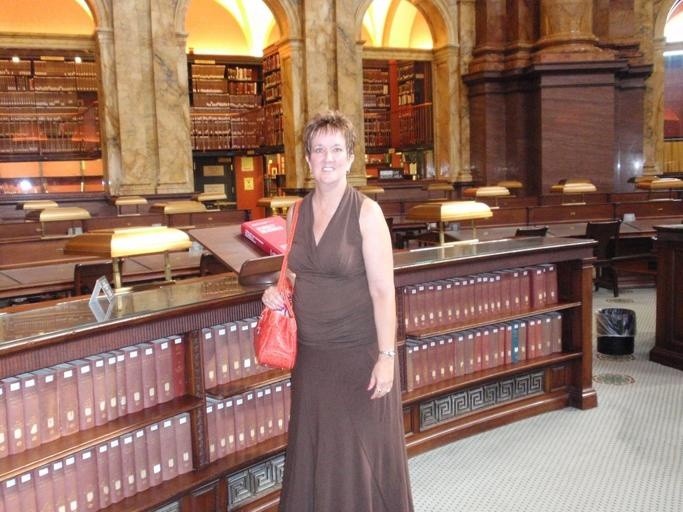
[261, 108, 415, 512]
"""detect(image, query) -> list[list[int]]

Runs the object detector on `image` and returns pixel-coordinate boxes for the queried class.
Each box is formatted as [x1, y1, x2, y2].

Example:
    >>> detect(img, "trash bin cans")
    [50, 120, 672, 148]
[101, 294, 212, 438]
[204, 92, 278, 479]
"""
[597, 309, 636, 355]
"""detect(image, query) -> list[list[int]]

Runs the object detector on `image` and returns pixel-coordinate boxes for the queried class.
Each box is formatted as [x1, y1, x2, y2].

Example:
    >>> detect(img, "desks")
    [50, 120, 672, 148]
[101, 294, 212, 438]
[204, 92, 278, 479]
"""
[359, 178, 682, 298]
[1, 196, 304, 299]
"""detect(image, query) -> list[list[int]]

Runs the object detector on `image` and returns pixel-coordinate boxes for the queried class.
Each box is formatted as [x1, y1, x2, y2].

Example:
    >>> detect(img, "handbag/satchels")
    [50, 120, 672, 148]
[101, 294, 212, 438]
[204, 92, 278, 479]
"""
[253, 277, 298, 371]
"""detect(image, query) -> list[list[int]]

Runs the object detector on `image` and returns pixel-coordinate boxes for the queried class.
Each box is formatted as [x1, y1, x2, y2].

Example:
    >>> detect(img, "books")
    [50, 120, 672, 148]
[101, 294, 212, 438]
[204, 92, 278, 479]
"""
[0, 55, 102, 157]
[184, 51, 287, 196]
[363, 62, 423, 150]
[239, 215, 288, 256]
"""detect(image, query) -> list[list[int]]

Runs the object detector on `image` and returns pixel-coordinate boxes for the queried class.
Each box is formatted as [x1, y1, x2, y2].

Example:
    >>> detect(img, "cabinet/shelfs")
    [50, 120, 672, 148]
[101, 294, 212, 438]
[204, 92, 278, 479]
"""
[363, 57, 434, 154]
[0, 238, 598, 512]
[0, 48, 103, 163]
[187, 51, 283, 156]
